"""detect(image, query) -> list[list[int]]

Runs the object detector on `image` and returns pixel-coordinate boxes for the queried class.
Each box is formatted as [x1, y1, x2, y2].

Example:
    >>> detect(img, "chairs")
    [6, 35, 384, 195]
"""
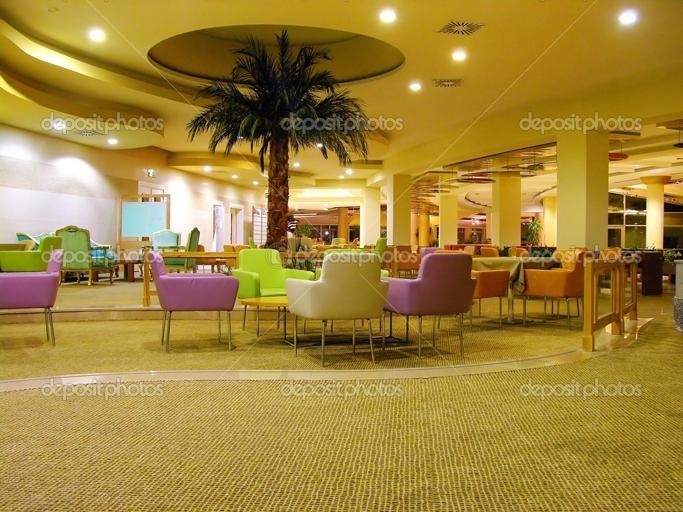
[0, 223, 683, 368]
